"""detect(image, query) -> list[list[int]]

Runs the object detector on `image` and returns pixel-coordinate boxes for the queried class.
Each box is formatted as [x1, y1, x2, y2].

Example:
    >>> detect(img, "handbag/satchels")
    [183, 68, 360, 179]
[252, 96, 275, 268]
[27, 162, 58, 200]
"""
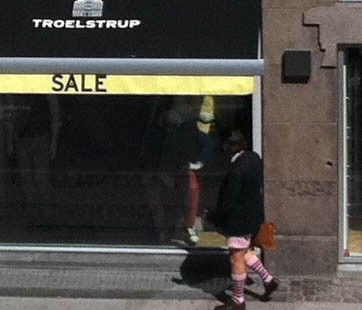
[252, 222, 277, 252]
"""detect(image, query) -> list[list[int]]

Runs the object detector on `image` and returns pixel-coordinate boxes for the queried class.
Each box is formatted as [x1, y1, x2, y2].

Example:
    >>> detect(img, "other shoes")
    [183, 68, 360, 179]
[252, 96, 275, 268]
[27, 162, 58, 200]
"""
[260, 278, 281, 302]
[184, 227, 199, 243]
[214, 298, 246, 310]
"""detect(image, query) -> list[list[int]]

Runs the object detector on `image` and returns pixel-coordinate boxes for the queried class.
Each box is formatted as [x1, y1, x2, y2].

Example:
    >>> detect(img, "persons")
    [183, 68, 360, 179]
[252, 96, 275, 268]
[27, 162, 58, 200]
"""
[211, 128, 282, 310]
[6, 93, 213, 245]
